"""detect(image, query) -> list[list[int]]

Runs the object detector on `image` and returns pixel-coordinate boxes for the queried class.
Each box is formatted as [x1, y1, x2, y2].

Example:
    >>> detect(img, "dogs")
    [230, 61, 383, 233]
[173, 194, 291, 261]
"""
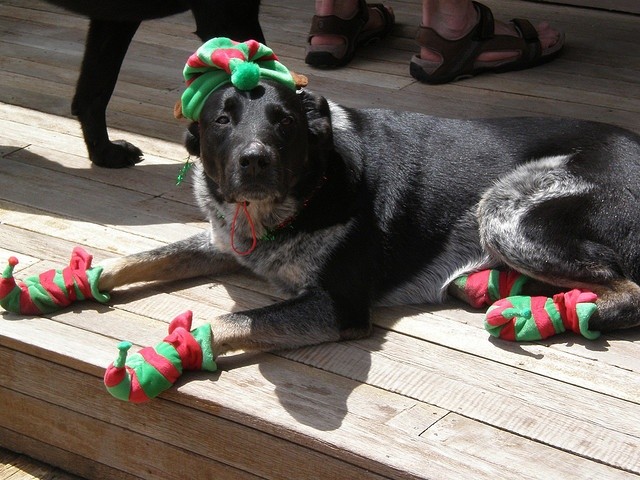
[0, 1, 271, 170]
[0, 37, 640, 403]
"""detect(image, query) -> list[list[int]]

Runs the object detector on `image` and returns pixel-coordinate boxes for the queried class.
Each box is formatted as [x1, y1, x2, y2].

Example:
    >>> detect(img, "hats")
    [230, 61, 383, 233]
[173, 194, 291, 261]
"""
[173, 36, 308, 121]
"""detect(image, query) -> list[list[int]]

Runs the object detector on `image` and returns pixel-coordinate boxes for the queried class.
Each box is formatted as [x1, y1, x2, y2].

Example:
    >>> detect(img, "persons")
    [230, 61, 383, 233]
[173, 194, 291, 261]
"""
[305, 0, 566, 84]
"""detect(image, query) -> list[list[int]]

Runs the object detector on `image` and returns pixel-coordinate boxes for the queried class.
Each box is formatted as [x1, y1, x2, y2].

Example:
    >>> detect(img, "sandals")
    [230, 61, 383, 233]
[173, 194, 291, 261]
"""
[411, 1, 565, 85]
[307, 0, 395, 68]
